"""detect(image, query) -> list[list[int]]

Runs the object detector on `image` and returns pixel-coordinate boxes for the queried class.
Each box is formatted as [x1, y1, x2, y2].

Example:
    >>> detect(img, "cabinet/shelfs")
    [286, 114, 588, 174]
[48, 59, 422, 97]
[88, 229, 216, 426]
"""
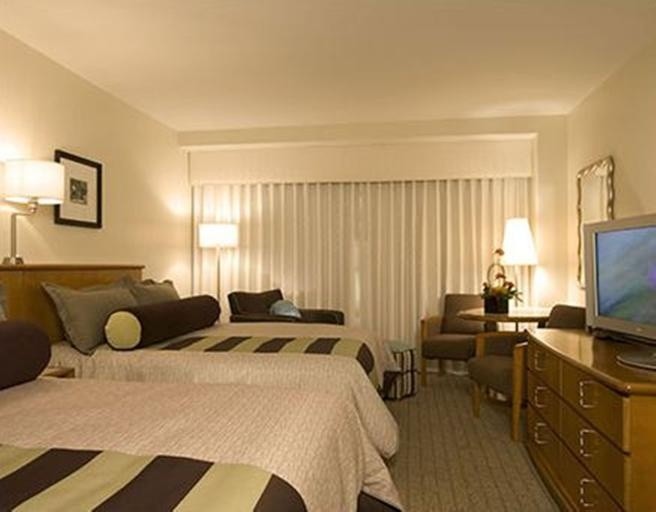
[523, 327, 561, 502]
[560, 359, 656, 512]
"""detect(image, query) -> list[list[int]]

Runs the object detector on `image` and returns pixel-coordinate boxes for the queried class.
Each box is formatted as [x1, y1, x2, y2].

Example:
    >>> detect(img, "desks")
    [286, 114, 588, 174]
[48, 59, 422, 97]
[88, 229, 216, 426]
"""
[456, 304, 552, 331]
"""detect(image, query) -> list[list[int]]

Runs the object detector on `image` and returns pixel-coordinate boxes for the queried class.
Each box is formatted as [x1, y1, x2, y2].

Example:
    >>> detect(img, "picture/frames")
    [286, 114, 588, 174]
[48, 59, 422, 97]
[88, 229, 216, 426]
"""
[54, 149, 103, 230]
[575, 155, 614, 290]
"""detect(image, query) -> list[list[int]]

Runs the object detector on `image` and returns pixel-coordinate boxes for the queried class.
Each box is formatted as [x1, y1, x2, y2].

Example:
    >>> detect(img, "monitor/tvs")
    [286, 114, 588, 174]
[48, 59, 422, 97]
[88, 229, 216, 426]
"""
[584, 213, 655, 371]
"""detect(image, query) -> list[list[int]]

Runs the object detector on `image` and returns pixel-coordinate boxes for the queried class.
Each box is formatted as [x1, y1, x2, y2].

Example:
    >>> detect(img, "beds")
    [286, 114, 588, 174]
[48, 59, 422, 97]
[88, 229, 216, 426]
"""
[0, 320, 407, 512]
[0, 263, 401, 461]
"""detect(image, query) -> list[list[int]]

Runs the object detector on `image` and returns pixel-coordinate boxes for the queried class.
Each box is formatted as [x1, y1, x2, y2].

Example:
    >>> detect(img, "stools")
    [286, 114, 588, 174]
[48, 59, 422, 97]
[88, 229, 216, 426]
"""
[386, 338, 419, 399]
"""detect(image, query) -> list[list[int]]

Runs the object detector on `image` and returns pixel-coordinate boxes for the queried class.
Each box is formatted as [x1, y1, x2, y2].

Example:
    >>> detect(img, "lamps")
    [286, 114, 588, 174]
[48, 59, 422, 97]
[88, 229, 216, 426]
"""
[0, 159, 65, 213]
[500, 217, 537, 265]
[198, 222, 239, 249]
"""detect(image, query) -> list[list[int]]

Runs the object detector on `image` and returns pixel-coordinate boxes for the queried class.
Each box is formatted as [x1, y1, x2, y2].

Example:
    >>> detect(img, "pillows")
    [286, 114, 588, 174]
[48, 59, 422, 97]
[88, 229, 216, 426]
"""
[40, 280, 138, 355]
[120, 274, 181, 306]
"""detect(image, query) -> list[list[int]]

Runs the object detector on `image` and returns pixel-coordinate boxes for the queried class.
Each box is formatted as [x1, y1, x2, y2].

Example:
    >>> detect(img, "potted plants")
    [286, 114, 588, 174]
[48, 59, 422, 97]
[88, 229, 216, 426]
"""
[480, 263, 524, 314]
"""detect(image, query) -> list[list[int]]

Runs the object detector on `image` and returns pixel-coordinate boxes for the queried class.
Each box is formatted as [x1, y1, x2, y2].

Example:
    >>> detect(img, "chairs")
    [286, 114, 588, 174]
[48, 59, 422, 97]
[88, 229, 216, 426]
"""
[419, 293, 486, 388]
[467, 304, 585, 441]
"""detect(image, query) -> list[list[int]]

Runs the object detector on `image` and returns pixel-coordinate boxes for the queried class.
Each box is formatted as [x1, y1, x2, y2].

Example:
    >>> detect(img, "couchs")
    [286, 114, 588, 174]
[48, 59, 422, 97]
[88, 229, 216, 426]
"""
[228, 288, 344, 324]
